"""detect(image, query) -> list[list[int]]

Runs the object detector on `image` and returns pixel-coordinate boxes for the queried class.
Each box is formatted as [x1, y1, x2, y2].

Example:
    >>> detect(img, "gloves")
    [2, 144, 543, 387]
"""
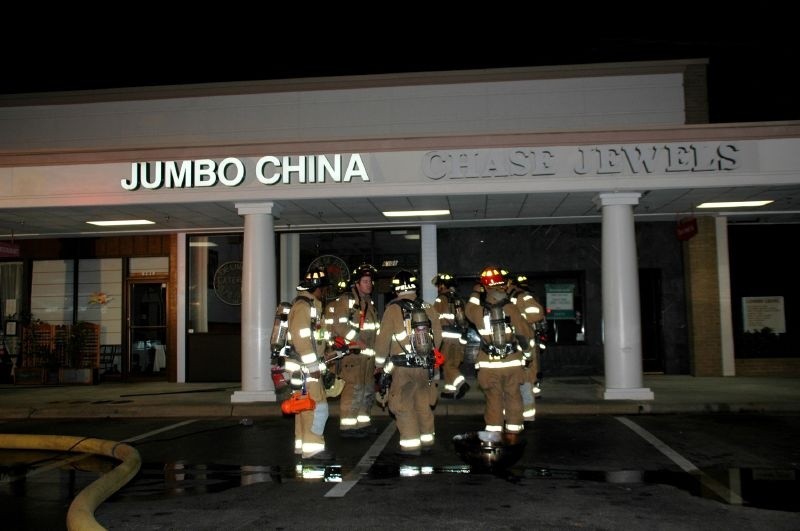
[310, 372, 320, 378]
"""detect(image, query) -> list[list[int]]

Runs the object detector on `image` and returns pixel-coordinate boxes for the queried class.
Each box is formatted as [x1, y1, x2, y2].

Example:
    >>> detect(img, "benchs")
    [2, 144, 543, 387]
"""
[99, 345, 117, 372]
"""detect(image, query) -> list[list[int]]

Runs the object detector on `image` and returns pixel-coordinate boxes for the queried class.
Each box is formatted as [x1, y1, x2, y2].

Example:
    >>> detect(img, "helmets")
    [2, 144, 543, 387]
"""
[435, 272, 456, 288]
[501, 270, 515, 283]
[480, 267, 505, 287]
[393, 270, 417, 292]
[515, 274, 536, 293]
[352, 264, 377, 278]
[295, 266, 338, 291]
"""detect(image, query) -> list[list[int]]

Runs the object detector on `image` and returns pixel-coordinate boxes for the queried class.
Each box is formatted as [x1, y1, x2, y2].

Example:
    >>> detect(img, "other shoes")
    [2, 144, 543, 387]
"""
[440, 391, 455, 400]
[340, 424, 379, 438]
[395, 447, 421, 459]
[505, 431, 516, 446]
[455, 383, 470, 399]
[303, 452, 336, 462]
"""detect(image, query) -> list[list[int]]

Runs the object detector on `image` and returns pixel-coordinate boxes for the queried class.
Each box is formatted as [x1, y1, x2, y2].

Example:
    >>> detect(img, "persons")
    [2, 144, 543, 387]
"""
[432, 273, 471, 400]
[374, 272, 445, 457]
[279, 263, 335, 462]
[325, 265, 381, 440]
[465, 266, 546, 447]
